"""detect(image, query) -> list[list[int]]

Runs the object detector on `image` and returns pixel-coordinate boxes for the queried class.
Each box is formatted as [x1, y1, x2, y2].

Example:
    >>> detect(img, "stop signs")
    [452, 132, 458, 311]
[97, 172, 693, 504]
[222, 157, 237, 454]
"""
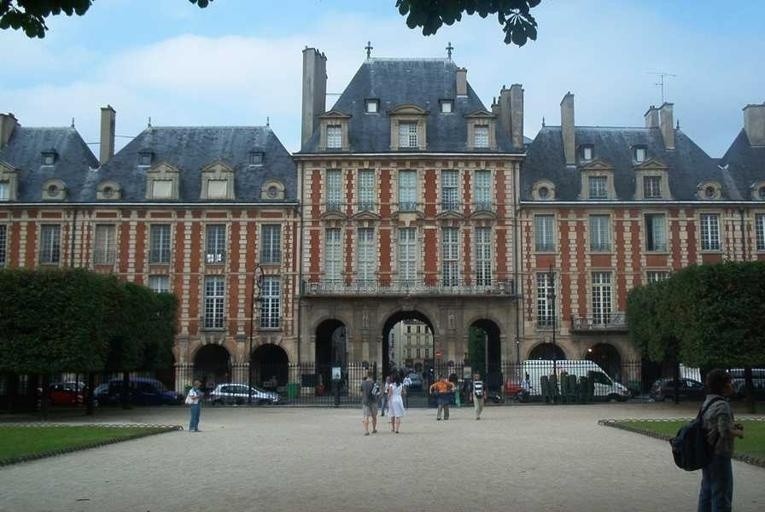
[435, 352, 440, 357]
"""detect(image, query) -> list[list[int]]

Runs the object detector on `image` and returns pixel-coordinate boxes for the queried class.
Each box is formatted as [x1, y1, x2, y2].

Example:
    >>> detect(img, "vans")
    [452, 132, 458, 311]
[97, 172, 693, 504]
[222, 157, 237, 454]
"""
[109, 377, 180, 406]
[727, 369, 765, 393]
[522, 359, 631, 403]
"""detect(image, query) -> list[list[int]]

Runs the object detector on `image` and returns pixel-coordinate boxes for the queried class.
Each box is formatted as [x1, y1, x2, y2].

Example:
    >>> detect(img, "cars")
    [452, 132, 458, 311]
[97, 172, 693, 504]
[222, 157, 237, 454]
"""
[429, 380, 500, 406]
[651, 377, 707, 402]
[389, 367, 423, 390]
[38, 383, 88, 408]
[210, 384, 280, 404]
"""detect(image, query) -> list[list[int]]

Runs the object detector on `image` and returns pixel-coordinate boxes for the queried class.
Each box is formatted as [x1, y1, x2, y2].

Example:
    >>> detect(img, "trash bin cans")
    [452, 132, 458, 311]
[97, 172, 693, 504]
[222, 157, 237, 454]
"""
[287, 383, 300, 400]
[628, 380, 641, 396]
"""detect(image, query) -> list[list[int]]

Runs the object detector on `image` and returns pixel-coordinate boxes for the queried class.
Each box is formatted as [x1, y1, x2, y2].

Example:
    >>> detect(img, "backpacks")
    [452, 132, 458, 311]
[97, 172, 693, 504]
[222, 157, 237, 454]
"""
[669, 397, 728, 471]
[371, 380, 383, 402]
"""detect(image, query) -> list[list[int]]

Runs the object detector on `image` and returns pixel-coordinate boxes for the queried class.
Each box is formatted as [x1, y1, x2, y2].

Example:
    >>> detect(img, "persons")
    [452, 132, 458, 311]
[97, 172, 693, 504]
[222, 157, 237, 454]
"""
[695, 369, 744, 511]
[358, 370, 413, 436]
[449, 373, 460, 408]
[187, 379, 205, 431]
[429, 373, 456, 420]
[520, 374, 534, 400]
[81, 383, 104, 408]
[468, 372, 488, 421]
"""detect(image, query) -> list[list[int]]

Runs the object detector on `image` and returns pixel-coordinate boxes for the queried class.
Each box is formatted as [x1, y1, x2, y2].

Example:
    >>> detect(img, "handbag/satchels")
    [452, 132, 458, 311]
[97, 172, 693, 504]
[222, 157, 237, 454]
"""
[475, 390, 483, 396]
[402, 386, 407, 397]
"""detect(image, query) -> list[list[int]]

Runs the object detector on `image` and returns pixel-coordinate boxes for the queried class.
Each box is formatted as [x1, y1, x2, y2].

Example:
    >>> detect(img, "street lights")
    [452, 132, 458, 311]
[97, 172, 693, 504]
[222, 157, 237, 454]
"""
[248, 266, 264, 404]
[547, 264, 556, 404]
